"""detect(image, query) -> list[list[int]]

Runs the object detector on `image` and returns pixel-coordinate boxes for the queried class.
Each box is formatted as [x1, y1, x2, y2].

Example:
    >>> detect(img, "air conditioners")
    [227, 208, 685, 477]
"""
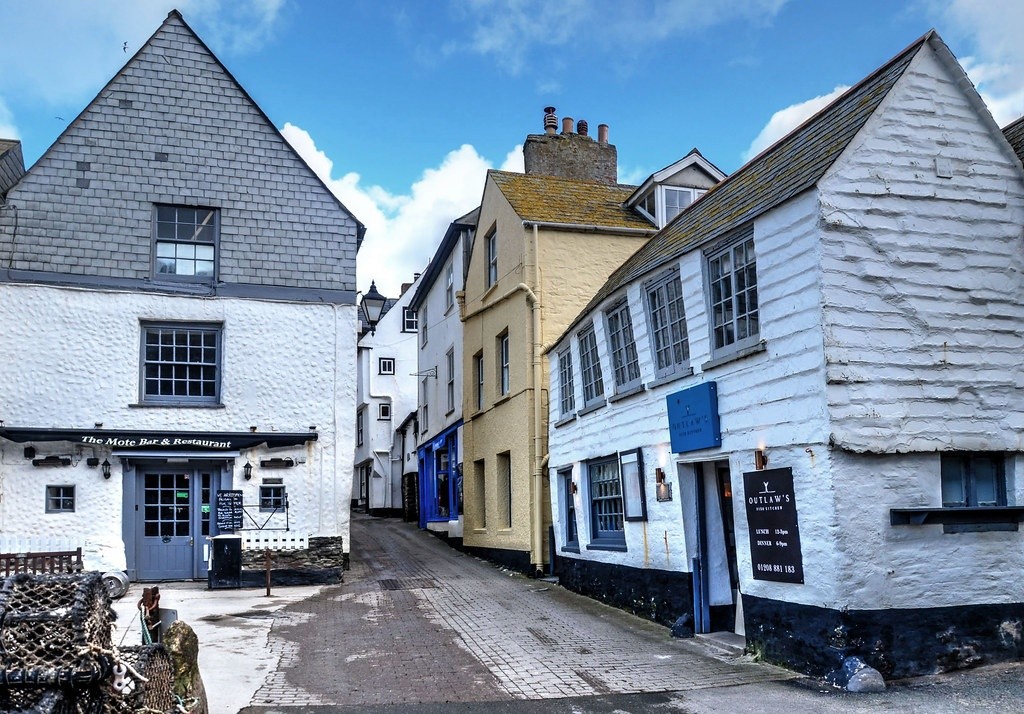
[243, 461, 252, 480]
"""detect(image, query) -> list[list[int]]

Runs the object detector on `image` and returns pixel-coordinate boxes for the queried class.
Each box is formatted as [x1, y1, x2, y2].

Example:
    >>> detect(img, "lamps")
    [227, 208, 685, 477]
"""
[102, 458, 111, 479]
[569, 482, 576, 494]
[655, 467, 665, 483]
[755, 451, 768, 470]
